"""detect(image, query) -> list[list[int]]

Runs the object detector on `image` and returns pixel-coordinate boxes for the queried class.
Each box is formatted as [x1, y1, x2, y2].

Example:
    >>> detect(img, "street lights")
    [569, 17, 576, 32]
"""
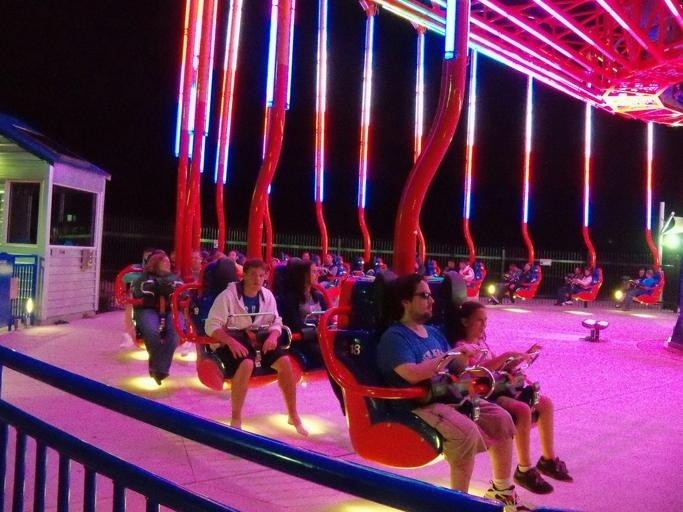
[654, 200, 681, 303]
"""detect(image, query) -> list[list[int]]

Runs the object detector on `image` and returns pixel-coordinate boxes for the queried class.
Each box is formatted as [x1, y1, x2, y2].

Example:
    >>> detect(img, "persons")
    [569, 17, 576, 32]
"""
[554, 266, 658, 311]
[133, 250, 575, 512]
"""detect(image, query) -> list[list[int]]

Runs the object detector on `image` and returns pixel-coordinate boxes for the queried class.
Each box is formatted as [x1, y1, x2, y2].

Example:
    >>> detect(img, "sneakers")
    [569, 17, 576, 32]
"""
[535, 454, 576, 484]
[148, 370, 170, 386]
[510, 463, 557, 495]
[482, 479, 531, 512]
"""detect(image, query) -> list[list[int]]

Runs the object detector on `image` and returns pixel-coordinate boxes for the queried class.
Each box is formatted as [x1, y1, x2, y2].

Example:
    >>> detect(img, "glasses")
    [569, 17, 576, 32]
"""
[412, 291, 432, 298]
